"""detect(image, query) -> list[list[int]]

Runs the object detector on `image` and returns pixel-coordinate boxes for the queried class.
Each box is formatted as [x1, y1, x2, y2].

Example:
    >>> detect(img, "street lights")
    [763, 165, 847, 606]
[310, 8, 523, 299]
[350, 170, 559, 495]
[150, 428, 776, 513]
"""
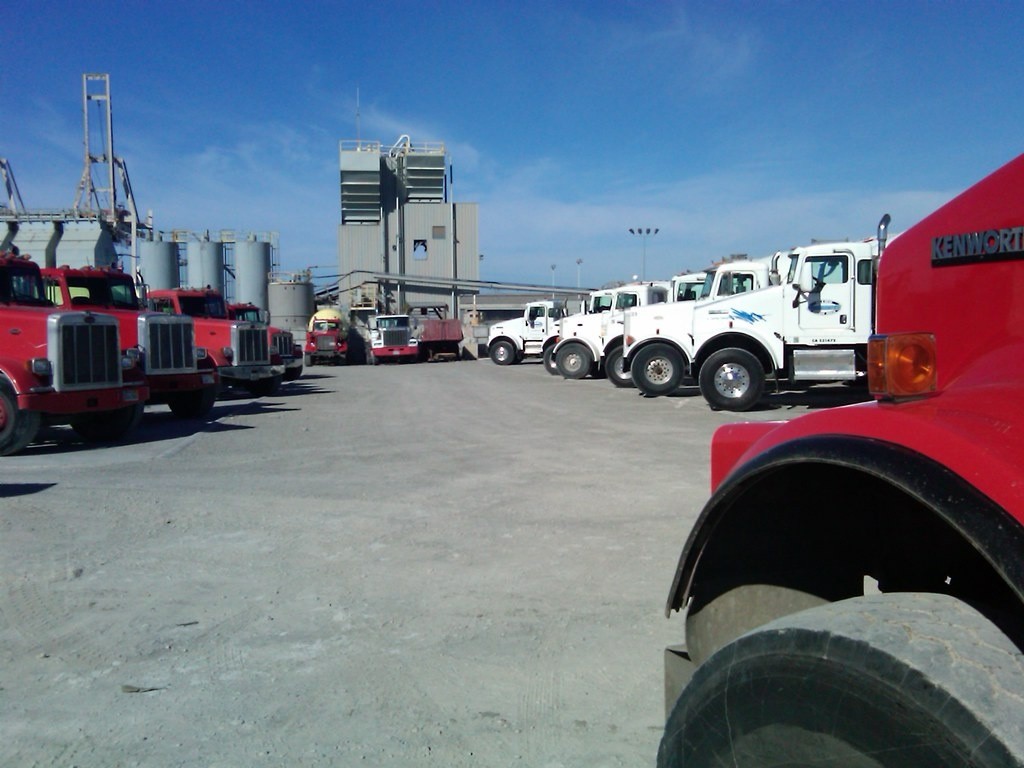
[551, 264, 557, 300]
[628, 227, 663, 280]
[575, 258, 583, 291]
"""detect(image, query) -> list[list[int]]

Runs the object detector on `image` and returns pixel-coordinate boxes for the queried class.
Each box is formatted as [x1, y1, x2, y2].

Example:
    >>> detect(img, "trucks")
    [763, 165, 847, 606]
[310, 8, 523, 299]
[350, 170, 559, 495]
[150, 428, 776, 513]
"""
[34, 264, 220, 417]
[228, 300, 309, 382]
[660, 142, 1024, 768]
[364, 315, 425, 364]
[141, 284, 291, 403]
[486, 211, 904, 413]
[1, 247, 153, 456]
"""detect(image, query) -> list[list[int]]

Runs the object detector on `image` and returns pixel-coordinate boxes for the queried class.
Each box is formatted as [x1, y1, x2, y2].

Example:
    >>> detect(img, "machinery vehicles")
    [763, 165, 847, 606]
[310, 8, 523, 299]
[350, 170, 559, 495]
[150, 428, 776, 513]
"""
[301, 309, 360, 367]
